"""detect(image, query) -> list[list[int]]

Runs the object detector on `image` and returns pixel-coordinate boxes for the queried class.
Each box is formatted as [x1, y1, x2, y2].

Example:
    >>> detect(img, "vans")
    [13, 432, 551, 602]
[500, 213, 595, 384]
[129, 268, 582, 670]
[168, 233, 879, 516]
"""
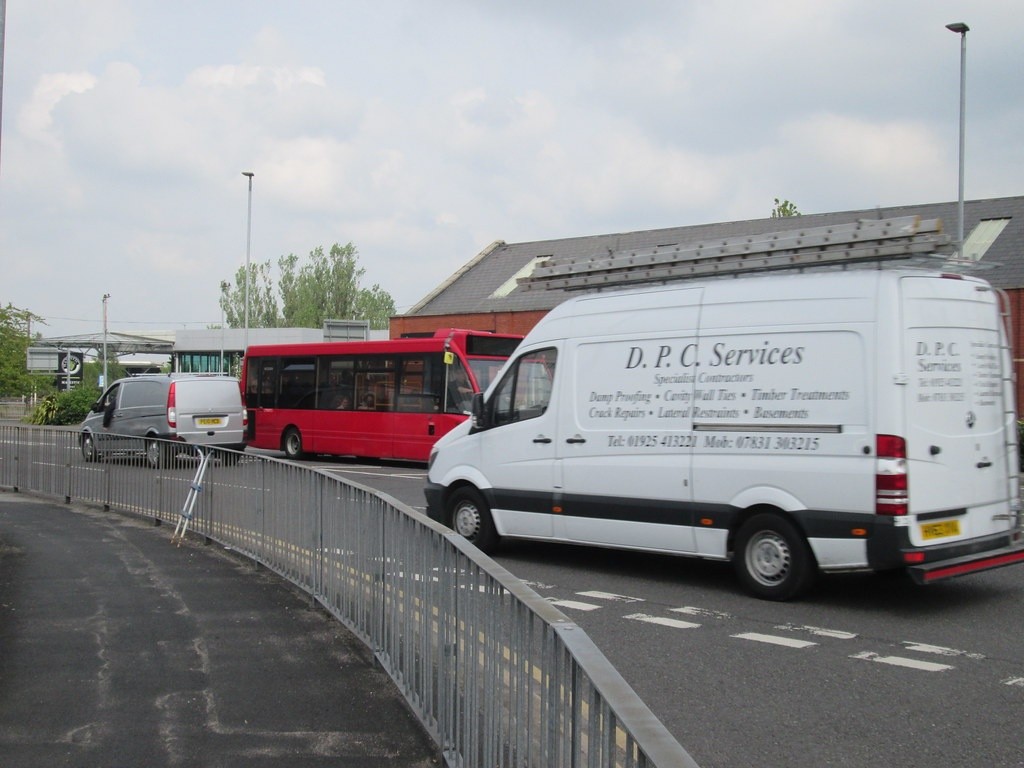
[422, 268, 1024, 605]
[77, 371, 249, 469]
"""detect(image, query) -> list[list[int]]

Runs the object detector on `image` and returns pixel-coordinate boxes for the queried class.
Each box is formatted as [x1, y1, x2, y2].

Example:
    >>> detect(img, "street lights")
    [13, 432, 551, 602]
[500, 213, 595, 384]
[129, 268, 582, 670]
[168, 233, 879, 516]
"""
[241, 171, 254, 328]
[945, 21, 971, 276]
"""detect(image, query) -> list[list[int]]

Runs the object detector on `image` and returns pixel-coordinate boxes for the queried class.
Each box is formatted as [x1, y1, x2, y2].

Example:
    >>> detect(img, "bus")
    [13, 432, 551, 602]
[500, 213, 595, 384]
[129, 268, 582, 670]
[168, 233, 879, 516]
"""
[241, 327, 554, 463]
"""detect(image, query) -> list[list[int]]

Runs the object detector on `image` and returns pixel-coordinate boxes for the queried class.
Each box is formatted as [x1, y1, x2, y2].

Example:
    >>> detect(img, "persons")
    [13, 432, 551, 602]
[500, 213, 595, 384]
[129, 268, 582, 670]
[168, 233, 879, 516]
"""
[453, 366, 474, 402]
[101, 398, 116, 432]
[358, 393, 376, 411]
[335, 396, 352, 410]
